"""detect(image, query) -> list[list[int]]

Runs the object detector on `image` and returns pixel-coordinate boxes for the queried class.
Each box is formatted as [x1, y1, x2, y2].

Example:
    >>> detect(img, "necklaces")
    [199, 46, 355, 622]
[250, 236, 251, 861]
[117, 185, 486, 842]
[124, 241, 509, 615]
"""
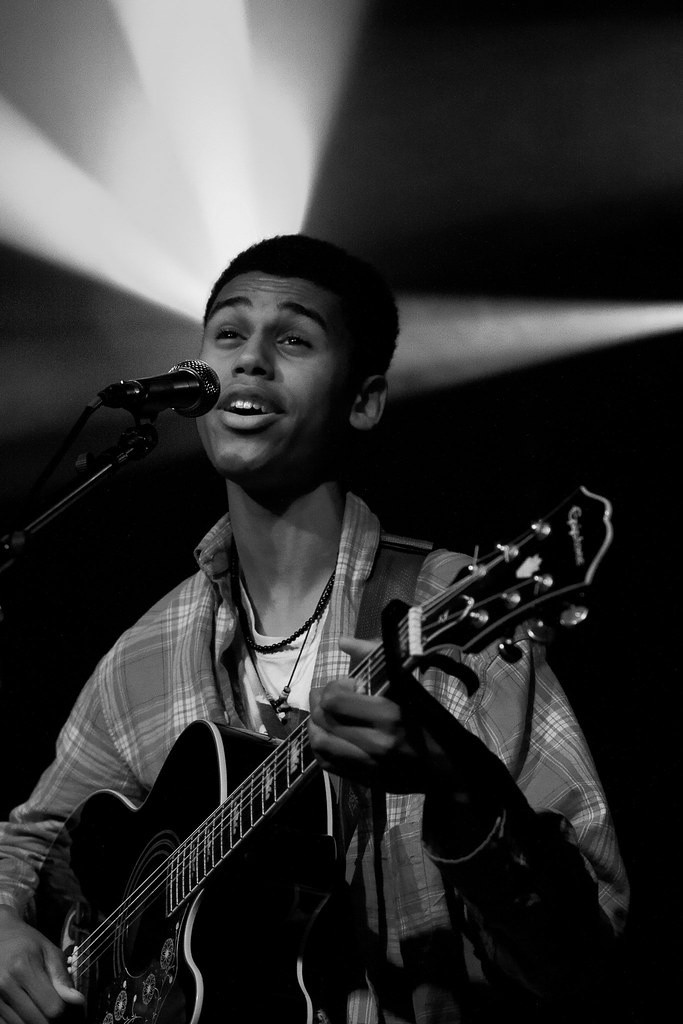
[230, 536, 338, 720]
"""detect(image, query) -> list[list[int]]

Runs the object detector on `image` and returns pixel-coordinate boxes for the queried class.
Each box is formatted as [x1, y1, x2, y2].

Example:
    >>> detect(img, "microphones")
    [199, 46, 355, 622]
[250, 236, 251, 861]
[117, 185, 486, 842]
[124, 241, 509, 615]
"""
[100, 360, 222, 418]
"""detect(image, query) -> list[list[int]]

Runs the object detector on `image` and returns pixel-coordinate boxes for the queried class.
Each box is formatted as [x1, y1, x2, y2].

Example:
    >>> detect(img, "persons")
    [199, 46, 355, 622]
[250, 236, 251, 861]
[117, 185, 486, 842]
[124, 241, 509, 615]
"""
[0, 235, 633, 1023]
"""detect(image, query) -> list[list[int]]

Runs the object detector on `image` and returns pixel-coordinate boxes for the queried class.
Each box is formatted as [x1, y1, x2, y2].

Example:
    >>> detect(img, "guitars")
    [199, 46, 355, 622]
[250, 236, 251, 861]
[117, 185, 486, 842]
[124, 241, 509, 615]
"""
[32, 481, 633, 1023]
